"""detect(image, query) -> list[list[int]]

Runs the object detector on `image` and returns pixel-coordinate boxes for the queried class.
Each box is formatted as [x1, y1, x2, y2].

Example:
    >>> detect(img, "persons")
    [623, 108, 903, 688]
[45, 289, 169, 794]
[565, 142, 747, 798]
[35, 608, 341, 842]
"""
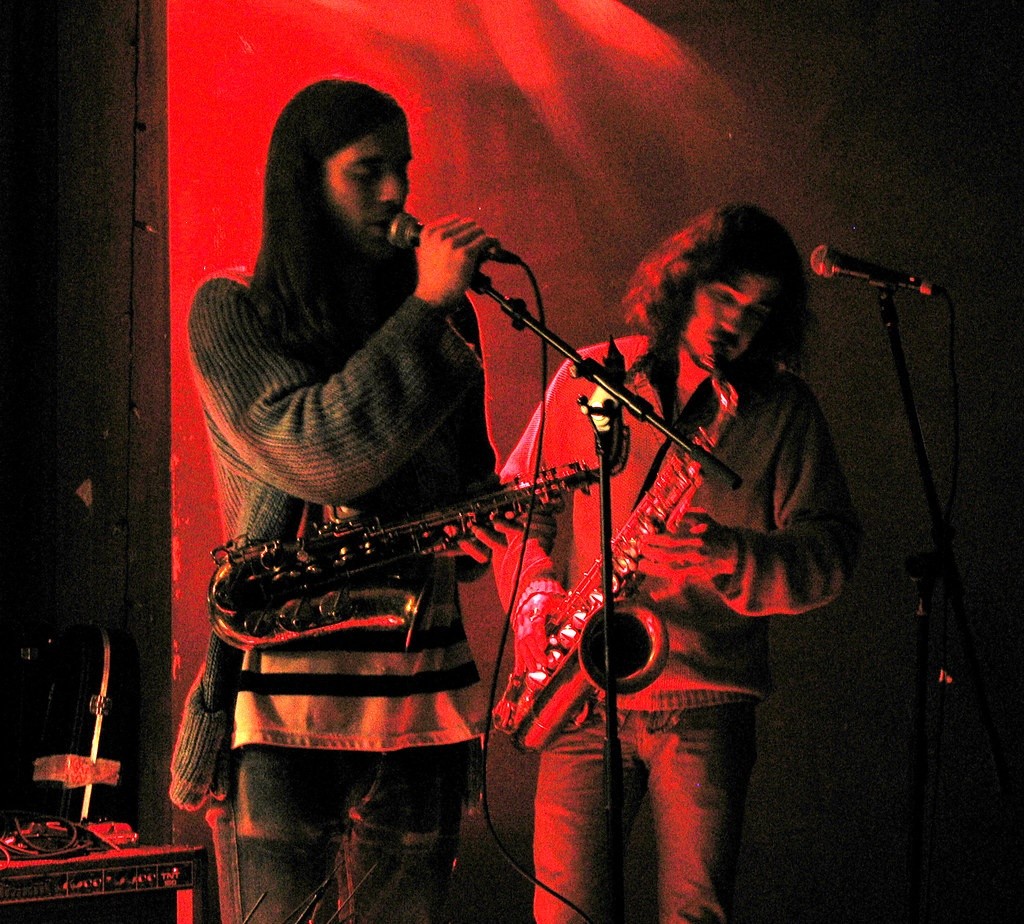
[494, 201, 859, 924]
[168, 80, 524, 924]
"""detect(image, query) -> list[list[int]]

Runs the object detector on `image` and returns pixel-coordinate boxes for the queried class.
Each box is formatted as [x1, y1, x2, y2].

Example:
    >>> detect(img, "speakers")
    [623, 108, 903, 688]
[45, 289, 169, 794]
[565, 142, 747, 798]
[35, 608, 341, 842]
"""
[1, 842, 211, 924]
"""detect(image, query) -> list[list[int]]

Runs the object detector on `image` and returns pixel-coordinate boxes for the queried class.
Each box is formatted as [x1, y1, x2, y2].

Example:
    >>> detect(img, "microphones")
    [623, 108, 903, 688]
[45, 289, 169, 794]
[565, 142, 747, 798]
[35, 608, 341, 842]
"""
[385, 211, 523, 266]
[809, 244, 944, 300]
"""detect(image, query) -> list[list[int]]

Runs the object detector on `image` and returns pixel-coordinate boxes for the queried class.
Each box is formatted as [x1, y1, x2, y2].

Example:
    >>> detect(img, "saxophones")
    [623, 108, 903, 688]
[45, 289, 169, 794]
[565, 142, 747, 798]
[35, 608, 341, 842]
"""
[489, 342, 754, 754]
[204, 333, 634, 657]
